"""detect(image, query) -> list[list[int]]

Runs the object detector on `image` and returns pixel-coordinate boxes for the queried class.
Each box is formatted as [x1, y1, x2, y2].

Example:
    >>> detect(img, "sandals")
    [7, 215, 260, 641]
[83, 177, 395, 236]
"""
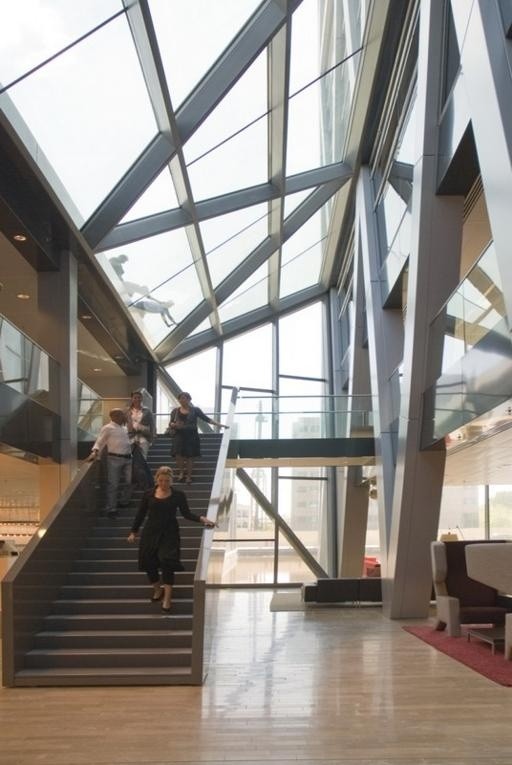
[151, 594, 171, 614]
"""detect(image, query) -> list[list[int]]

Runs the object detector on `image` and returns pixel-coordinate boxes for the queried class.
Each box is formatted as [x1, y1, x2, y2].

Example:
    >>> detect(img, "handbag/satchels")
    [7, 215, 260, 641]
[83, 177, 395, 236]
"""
[167, 428, 176, 437]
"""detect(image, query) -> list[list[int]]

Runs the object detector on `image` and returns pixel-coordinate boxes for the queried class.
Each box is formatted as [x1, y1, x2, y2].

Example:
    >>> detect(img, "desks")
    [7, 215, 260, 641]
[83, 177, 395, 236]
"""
[300, 584, 318, 602]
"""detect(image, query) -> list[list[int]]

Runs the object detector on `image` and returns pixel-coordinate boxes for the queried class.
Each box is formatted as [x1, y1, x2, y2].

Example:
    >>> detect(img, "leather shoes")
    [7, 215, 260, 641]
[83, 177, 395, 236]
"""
[109, 505, 130, 518]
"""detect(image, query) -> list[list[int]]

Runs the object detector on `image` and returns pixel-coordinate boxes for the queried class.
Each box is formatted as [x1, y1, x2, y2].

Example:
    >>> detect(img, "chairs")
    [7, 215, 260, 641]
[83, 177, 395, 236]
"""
[465, 543, 512, 660]
[430, 539, 512, 637]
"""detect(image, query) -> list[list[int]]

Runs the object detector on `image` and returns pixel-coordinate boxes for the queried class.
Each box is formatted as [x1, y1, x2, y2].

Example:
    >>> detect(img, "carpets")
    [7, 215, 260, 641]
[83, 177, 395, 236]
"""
[403, 625, 512, 687]
[270, 593, 383, 611]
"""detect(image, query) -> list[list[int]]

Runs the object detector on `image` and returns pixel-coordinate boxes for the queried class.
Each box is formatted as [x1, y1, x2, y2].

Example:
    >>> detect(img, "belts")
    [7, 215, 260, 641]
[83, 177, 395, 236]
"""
[108, 453, 131, 459]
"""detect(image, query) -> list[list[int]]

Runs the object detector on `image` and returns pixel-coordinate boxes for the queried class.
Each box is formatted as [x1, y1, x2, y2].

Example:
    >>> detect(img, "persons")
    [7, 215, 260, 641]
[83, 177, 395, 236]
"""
[168, 392, 229, 482]
[127, 466, 219, 615]
[81, 392, 155, 517]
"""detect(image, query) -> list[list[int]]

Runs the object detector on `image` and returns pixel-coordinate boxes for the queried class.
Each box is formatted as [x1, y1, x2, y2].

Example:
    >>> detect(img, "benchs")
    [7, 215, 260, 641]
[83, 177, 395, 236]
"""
[316, 579, 382, 608]
